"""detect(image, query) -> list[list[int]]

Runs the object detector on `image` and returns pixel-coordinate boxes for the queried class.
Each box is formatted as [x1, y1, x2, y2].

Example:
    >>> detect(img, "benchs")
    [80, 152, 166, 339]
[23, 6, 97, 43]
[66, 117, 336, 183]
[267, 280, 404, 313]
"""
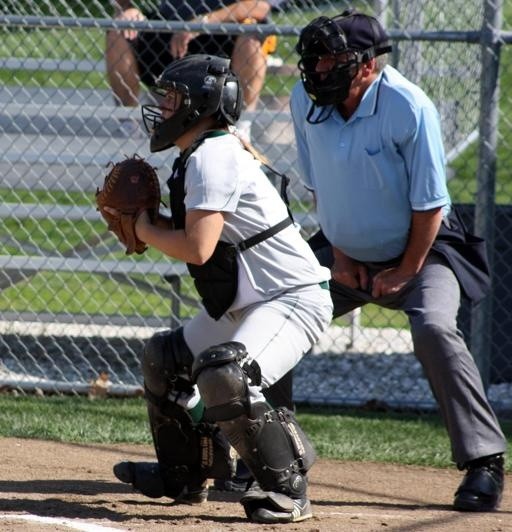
[0, 55, 475, 408]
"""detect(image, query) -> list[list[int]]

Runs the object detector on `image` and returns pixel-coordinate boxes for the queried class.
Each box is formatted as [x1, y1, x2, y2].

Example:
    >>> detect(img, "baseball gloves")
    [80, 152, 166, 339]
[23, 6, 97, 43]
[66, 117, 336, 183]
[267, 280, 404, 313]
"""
[95, 153, 167, 255]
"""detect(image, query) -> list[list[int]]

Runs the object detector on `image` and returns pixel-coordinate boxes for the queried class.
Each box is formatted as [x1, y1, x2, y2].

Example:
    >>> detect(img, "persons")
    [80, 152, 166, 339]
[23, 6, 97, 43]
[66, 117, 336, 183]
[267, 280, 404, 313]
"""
[200, 12, 511, 515]
[94, 49, 336, 524]
[101, 1, 286, 146]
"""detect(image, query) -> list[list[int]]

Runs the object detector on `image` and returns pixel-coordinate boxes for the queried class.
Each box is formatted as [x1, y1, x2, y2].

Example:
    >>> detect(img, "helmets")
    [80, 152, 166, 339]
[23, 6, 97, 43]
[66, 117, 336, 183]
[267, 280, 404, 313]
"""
[141, 53, 241, 153]
[297, 8, 392, 107]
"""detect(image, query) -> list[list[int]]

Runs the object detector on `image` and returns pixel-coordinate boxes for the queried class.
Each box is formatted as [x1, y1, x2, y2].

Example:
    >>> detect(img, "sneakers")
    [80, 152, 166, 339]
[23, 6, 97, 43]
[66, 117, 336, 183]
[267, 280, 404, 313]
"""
[250, 493, 313, 524]
[452, 453, 503, 510]
[225, 469, 255, 492]
[133, 473, 208, 504]
